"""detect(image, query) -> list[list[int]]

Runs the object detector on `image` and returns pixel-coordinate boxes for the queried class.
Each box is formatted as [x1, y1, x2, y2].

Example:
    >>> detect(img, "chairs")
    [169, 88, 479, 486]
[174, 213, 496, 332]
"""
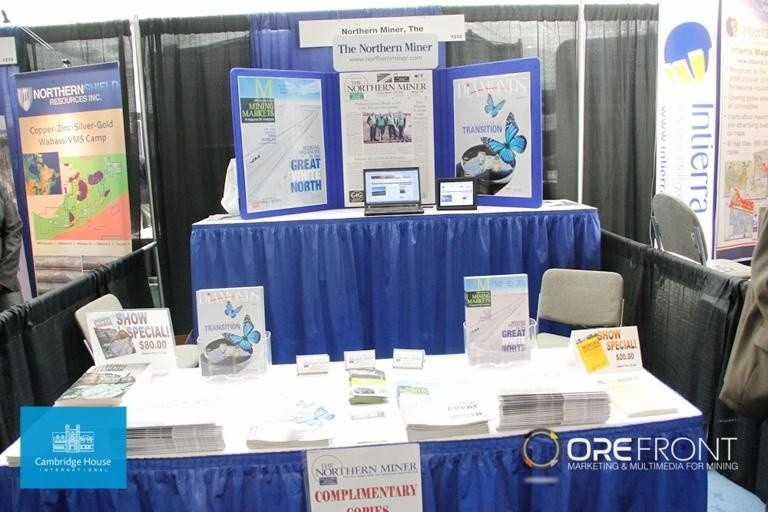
[74, 293, 123, 365]
[650, 192, 752, 279]
[536, 268, 624, 349]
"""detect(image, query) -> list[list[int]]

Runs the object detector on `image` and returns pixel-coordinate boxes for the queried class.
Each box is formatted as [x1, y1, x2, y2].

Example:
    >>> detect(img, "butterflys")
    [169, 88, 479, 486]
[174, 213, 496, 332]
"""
[223, 314, 262, 354]
[224, 300, 244, 319]
[479, 111, 528, 163]
[483, 94, 505, 118]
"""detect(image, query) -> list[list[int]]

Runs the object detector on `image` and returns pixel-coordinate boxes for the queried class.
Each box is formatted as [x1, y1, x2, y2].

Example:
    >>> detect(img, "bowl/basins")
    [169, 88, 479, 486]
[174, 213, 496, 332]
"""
[203, 337, 254, 373]
[461, 144, 518, 195]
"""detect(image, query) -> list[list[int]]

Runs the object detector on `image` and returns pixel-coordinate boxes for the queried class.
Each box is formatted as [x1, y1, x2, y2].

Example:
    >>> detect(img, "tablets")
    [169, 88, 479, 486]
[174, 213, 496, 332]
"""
[435, 177, 477, 210]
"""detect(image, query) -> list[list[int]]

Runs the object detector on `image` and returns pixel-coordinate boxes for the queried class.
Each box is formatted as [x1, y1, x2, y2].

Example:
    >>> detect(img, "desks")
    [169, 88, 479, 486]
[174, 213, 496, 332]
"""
[190, 199, 598, 363]
[0, 346, 707, 512]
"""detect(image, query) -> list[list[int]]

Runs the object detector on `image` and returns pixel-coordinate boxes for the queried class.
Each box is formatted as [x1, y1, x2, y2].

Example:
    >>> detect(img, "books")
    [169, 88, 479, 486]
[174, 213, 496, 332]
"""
[8, 271, 678, 467]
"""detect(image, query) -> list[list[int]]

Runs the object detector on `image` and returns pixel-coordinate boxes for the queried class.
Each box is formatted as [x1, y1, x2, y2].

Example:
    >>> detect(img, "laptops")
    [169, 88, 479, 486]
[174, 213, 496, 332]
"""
[362, 167, 424, 216]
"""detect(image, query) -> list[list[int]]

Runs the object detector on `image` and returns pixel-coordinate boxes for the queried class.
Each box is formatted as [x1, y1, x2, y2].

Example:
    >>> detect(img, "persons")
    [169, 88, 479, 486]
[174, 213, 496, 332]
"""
[1, 177, 30, 313]
[367, 110, 406, 142]
